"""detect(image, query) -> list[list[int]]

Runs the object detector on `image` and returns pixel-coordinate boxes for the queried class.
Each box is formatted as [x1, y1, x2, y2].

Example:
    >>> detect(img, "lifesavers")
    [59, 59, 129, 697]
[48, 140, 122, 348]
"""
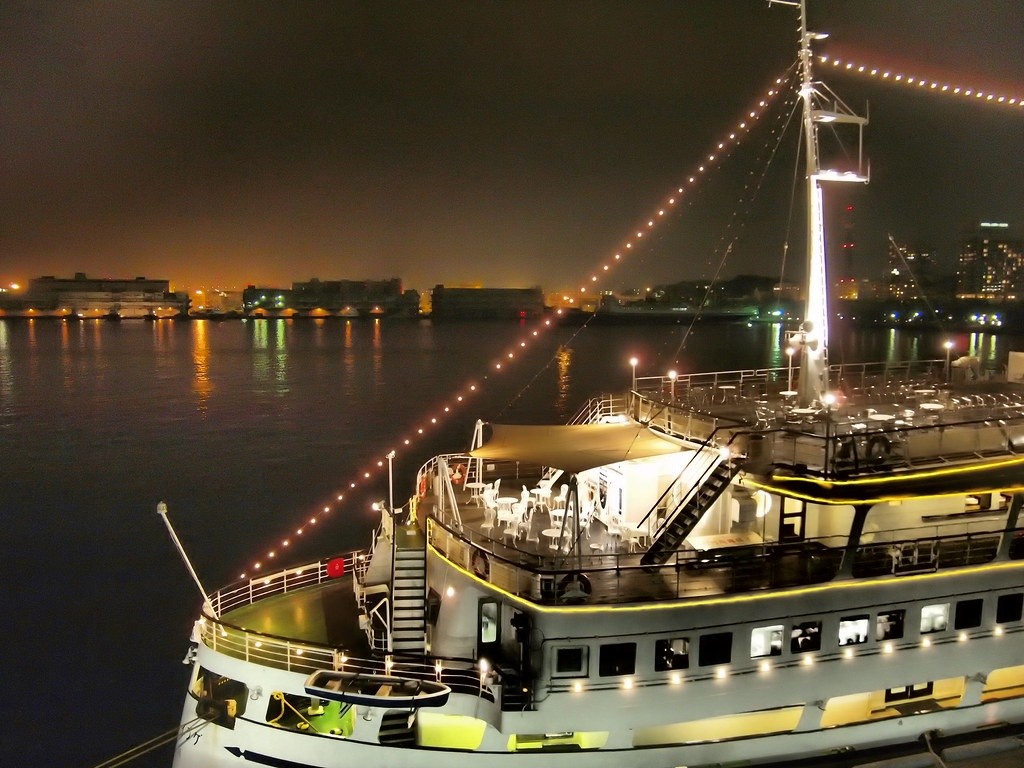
[471, 551, 493, 579]
[451, 462, 466, 485]
[866, 434, 891, 462]
[557, 573, 594, 603]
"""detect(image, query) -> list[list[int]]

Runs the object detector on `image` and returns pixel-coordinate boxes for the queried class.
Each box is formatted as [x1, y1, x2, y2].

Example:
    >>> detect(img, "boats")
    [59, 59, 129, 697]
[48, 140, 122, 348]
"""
[161, 0, 1024, 768]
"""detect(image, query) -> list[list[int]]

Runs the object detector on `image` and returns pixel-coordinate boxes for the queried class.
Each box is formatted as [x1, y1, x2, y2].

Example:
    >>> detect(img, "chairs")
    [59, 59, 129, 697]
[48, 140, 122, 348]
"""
[640, 371, 1024, 448]
[464, 477, 666, 566]
